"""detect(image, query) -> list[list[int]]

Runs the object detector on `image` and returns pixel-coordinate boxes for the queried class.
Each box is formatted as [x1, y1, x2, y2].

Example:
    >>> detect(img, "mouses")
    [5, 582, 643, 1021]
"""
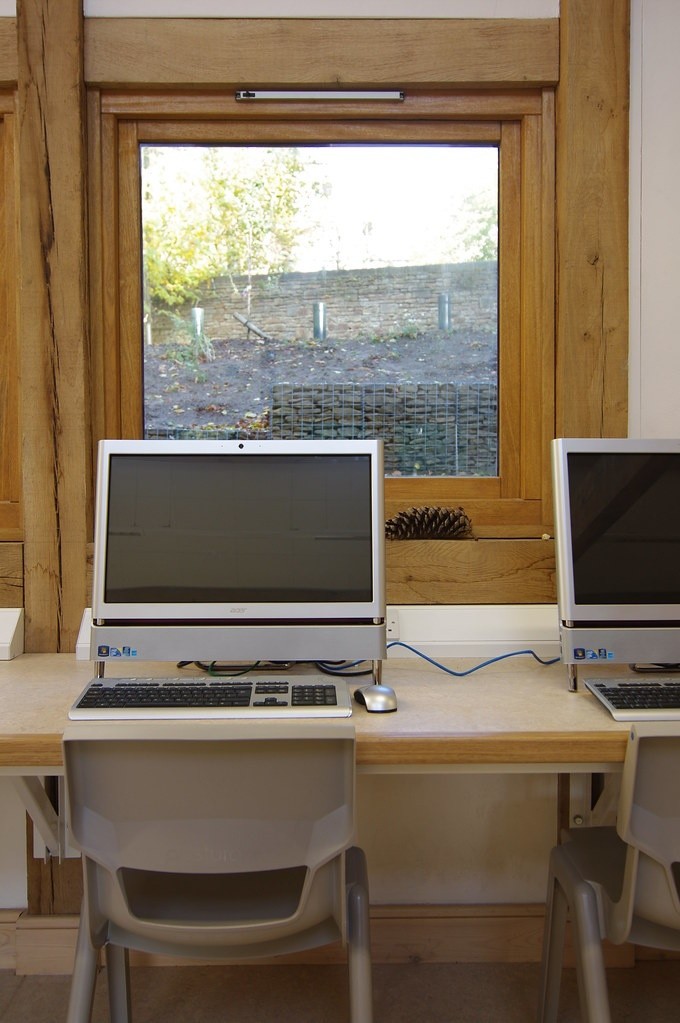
[354, 685, 397, 713]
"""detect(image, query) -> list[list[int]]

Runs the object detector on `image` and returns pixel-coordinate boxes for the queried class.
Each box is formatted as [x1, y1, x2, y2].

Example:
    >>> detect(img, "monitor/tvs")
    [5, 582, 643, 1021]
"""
[550, 438, 680, 693]
[88, 440, 388, 689]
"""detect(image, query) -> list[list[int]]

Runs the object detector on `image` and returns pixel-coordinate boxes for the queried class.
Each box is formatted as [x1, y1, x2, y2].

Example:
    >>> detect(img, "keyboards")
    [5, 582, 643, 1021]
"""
[66, 676, 353, 720]
[580, 677, 680, 722]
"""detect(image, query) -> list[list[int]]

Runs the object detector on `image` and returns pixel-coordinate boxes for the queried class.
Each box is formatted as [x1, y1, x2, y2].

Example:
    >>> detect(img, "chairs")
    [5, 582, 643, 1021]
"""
[60, 724, 373, 1023]
[537, 720, 680, 1023]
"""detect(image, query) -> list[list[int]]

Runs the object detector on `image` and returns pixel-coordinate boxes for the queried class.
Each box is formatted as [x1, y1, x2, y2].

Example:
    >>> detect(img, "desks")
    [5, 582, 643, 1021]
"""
[0, 652, 680, 853]
